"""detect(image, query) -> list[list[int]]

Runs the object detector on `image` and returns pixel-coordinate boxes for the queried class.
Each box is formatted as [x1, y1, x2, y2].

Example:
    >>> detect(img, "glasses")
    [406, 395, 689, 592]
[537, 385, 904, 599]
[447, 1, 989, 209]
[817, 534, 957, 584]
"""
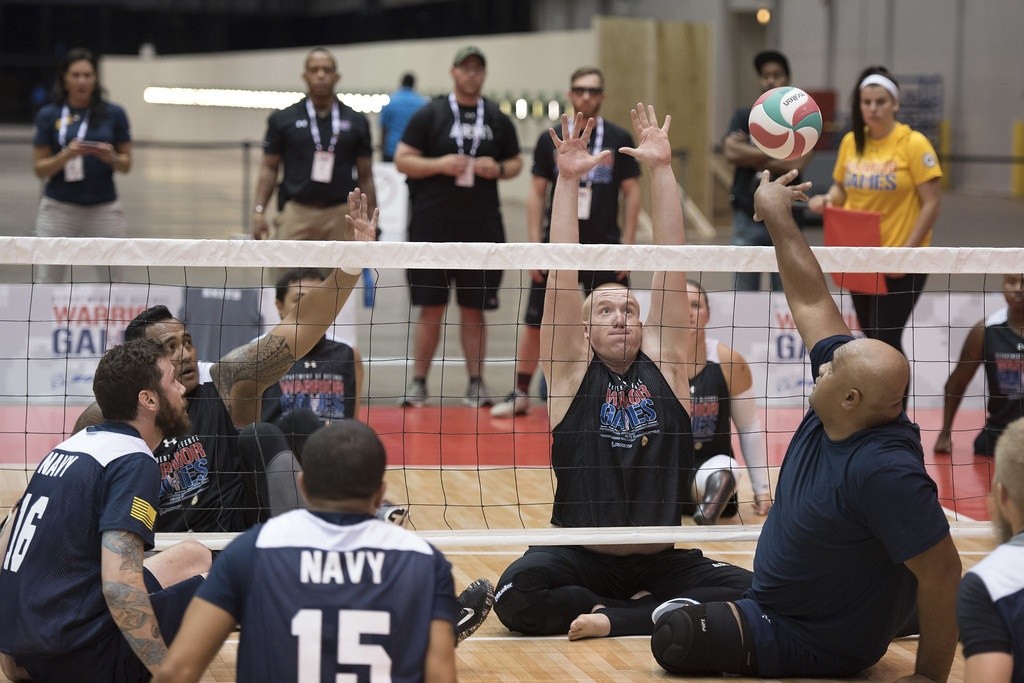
[571, 87, 603, 95]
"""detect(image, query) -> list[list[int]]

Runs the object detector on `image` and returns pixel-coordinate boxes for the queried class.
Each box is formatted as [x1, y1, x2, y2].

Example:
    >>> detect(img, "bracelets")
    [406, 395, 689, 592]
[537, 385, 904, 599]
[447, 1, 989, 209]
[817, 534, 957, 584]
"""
[498, 161, 505, 179]
[255, 205, 264, 213]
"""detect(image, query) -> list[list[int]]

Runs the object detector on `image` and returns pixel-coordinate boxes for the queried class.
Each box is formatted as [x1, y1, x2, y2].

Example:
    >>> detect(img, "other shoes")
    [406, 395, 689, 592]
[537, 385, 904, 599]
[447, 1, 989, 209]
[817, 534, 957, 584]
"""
[651, 598, 702, 624]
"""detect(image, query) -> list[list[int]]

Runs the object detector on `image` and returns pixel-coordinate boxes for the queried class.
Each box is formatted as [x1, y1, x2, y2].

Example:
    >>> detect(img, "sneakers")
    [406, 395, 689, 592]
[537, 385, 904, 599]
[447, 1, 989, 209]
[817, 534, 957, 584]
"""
[455, 579, 494, 646]
[489, 389, 529, 416]
[397, 384, 426, 406]
[462, 383, 494, 407]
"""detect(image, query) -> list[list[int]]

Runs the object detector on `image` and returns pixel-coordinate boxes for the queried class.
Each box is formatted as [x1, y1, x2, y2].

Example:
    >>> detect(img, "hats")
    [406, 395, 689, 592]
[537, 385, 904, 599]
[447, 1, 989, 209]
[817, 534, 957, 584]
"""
[454, 46, 485, 67]
[754, 51, 790, 76]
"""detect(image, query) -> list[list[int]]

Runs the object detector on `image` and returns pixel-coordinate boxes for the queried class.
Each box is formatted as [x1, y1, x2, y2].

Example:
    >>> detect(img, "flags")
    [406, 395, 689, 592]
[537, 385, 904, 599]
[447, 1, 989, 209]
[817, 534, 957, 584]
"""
[822, 206, 886, 294]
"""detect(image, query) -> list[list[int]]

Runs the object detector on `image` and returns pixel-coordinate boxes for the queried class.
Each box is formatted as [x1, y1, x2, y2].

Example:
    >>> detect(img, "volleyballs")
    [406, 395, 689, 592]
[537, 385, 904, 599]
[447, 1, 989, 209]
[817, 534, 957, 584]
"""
[748, 86, 824, 161]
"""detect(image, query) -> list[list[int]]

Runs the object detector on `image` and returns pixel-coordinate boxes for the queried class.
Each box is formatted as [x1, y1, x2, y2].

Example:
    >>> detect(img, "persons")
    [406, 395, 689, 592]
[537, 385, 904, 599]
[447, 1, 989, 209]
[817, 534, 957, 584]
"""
[149, 419, 458, 683]
[31, 49, 134, 281]
[252, 49, 378, 283]
[70, 187, 379, 532]
[956, 418, 1024, 683]
[393, 47, 523, 407]
[493, 104, 755, 640]
[650, 169, 963, 683]
[807, 67, 945, 413]
[0, 340, 494, 683]
[723, 47, 812, 290]
[378, 74, 433, 162]
[490, 67, 643, 417]
[250, 268, 411, 528]
[684, 278, 772, 525]
[934, 274, 1024, 456]
